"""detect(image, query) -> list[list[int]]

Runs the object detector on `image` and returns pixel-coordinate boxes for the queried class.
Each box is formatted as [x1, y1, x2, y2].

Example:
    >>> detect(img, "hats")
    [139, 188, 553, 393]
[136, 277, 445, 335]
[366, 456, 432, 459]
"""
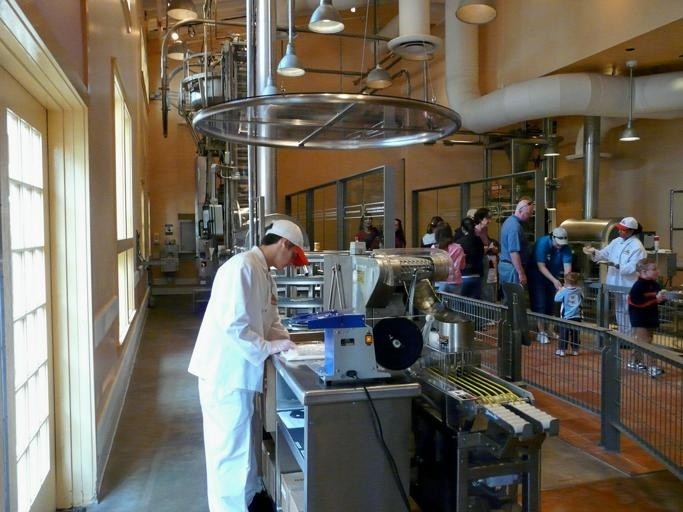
[615, 216, 638, 231]
[265, 219, 310, 267]
[553, 227, 569, 245]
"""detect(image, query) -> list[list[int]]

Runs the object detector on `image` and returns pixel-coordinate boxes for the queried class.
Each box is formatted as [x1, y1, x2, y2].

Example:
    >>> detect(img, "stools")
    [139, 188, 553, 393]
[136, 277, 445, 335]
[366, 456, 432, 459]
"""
[192, 286, 211, 314]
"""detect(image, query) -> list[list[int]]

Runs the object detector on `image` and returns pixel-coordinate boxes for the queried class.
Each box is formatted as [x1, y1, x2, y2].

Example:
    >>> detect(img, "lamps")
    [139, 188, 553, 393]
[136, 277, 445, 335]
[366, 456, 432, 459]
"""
[306, 1, 347, 35]
[366, 38, 396, 90]
[454, 1, 497, 26]
[277, 2, 309, 79]
[164, 37, 194, 63]
[543, 60, 641, 157]
[166, 2, 201, 23]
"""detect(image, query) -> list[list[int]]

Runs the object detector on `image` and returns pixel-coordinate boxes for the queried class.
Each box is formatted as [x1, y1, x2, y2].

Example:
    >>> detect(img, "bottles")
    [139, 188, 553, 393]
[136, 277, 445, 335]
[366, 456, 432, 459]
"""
[654, 236, 660, 250]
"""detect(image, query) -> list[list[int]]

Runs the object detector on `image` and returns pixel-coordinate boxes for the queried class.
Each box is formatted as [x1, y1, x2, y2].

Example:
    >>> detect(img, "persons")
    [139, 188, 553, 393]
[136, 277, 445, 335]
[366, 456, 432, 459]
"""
[628, 258, 666, 378]
[395, 218, 406, 249]
[582, 217, 647, 349]
[186, 221, 307, 511]
[355, 212, 382, 250]
[553, 272, 584, 356]
[422, 199, 572, 344]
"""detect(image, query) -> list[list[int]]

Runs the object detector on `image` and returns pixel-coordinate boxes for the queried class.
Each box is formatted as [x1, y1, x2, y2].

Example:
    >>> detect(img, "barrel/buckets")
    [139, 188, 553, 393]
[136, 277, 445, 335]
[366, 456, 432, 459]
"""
[428, 326, 439, 347]
[438, 319, 474, 352]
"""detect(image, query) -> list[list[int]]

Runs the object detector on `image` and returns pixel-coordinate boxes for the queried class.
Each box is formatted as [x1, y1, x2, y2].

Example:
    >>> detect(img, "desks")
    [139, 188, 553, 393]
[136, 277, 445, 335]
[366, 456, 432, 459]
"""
[262, 340, 423, 512]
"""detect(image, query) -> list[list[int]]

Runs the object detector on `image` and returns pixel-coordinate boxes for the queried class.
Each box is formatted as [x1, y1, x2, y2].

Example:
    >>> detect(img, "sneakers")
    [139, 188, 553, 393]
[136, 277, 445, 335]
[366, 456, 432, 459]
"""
[628, 362, 664, 377]
[521, 329, 580, 356]
